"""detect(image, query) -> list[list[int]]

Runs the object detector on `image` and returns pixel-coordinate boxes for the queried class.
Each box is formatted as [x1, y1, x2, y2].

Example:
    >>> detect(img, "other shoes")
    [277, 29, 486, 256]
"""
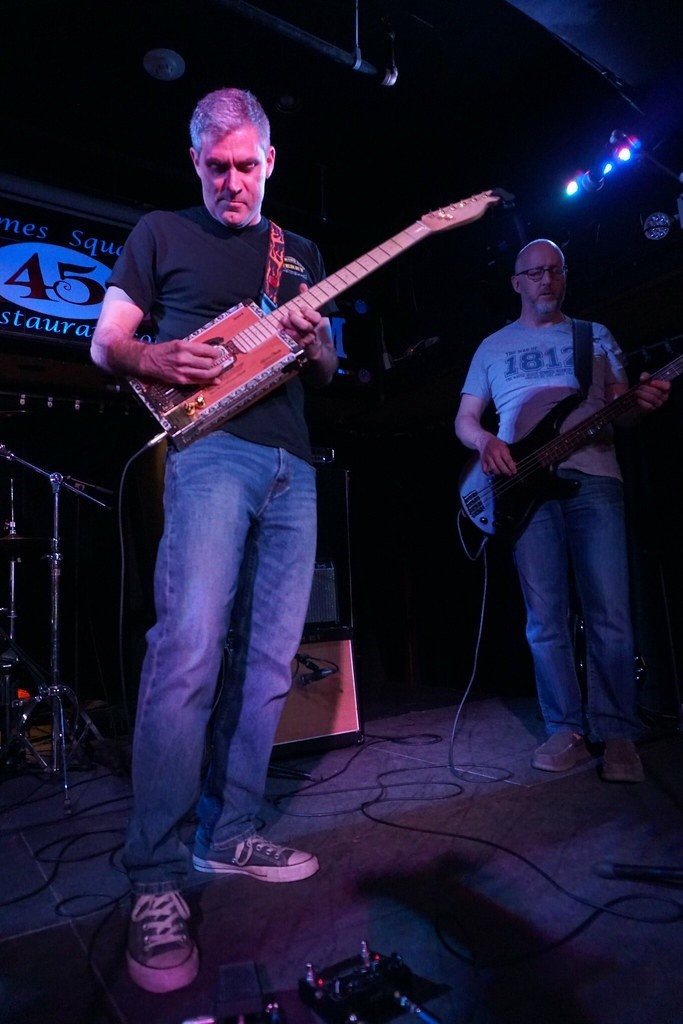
[533, 728, 592, 773]
[602, 740, 643, 783]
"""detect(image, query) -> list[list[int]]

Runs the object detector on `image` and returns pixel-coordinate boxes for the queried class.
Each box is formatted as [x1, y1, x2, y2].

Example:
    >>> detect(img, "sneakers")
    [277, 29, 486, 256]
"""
[191, 831, 318, 884]
[125, 888, 200, 995]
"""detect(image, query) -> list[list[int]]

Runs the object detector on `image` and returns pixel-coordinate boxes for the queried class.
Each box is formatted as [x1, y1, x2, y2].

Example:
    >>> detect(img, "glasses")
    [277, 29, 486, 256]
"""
[516, 266, 567, 283]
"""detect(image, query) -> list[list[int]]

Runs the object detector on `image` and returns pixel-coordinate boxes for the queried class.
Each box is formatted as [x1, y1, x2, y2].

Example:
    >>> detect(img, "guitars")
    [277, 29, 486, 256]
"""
[453, 349, 682, 567]
[127, 182, 506, 444]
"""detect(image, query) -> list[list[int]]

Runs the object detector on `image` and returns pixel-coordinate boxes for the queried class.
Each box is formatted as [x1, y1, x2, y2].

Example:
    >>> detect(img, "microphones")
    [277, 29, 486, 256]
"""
[297, 668, 338, 688]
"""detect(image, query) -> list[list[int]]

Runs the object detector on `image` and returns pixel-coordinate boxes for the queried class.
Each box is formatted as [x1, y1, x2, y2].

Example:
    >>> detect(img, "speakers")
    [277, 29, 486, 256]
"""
[271, 625, 360, 748]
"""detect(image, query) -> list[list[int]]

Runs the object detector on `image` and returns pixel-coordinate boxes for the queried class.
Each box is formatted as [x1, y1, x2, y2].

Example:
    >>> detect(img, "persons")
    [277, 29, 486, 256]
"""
[89, 88, 350, 994]
[456, 237, 670, 784]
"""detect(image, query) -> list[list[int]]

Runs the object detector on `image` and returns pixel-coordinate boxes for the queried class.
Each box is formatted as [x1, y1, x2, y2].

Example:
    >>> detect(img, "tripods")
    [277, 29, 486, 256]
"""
[0, 446, 127, 817]
